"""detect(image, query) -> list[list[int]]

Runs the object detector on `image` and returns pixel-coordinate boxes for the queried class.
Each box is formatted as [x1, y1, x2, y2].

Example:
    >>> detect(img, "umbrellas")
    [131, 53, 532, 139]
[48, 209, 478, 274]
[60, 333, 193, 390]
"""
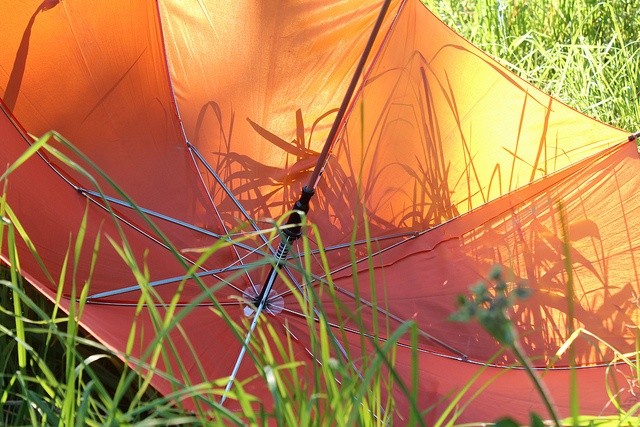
[0, 1, 639, 427]
